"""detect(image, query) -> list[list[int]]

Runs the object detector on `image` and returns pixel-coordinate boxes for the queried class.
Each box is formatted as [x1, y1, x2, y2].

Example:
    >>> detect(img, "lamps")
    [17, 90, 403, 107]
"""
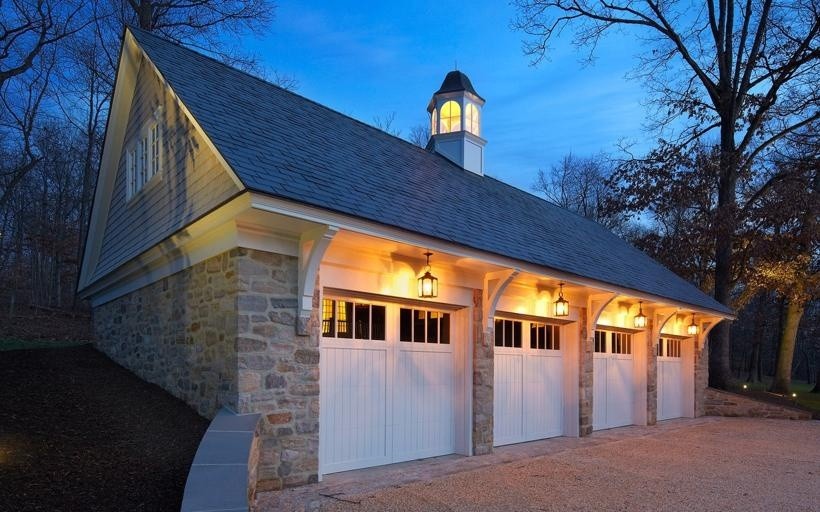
[687, 312, 700, 335]
[634, 301, 648, 328]
[417, 250, 440, 301]
[553, 280, 570, 317]
